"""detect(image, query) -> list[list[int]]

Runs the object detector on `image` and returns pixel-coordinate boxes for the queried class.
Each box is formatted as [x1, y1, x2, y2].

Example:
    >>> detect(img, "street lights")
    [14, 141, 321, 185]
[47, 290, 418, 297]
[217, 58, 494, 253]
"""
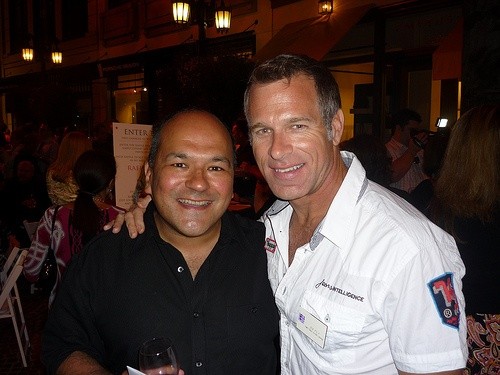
[20, 29, 63, 84]
[171, 0, 233, 57]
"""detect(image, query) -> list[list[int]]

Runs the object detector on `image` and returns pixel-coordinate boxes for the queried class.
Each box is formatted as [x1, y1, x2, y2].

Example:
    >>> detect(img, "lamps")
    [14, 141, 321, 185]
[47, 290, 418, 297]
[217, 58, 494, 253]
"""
[318, 0, 332, 13]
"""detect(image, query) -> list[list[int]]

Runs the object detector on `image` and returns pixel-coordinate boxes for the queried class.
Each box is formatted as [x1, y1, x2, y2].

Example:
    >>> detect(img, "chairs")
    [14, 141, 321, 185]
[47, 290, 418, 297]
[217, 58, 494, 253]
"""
[0, 247, 31, 367]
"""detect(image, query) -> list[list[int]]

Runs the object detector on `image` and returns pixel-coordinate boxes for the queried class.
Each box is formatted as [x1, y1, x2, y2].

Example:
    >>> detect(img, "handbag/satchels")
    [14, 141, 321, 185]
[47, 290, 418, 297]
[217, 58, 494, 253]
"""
[36, 204, 58, 294]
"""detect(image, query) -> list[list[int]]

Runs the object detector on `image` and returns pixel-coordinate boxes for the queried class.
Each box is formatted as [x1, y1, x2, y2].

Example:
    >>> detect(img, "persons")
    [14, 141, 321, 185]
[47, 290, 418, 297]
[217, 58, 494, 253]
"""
[45, 131, 95, 204]
[103, 53, 469, 375]
[231, 119, 258, 206]
[0, 159, 52, 248]
[0, 119, 122, 185]
[239, 160, 273, 214]
[21, 148, 128, 325]
[382, 108, 434, 215]
[425, 101, 500, 375]
[39, 103, 283, 375]
[336, 133, 410, 202]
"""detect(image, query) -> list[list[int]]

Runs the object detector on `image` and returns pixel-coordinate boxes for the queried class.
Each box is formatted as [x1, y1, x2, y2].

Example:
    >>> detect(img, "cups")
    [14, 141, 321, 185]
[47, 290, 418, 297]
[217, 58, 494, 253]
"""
[139, 336, 179, 375]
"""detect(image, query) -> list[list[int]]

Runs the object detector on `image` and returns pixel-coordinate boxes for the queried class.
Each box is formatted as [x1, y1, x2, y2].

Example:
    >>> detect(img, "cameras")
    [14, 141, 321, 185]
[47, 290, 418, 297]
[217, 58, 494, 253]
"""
[410, 127, 437, 149]
